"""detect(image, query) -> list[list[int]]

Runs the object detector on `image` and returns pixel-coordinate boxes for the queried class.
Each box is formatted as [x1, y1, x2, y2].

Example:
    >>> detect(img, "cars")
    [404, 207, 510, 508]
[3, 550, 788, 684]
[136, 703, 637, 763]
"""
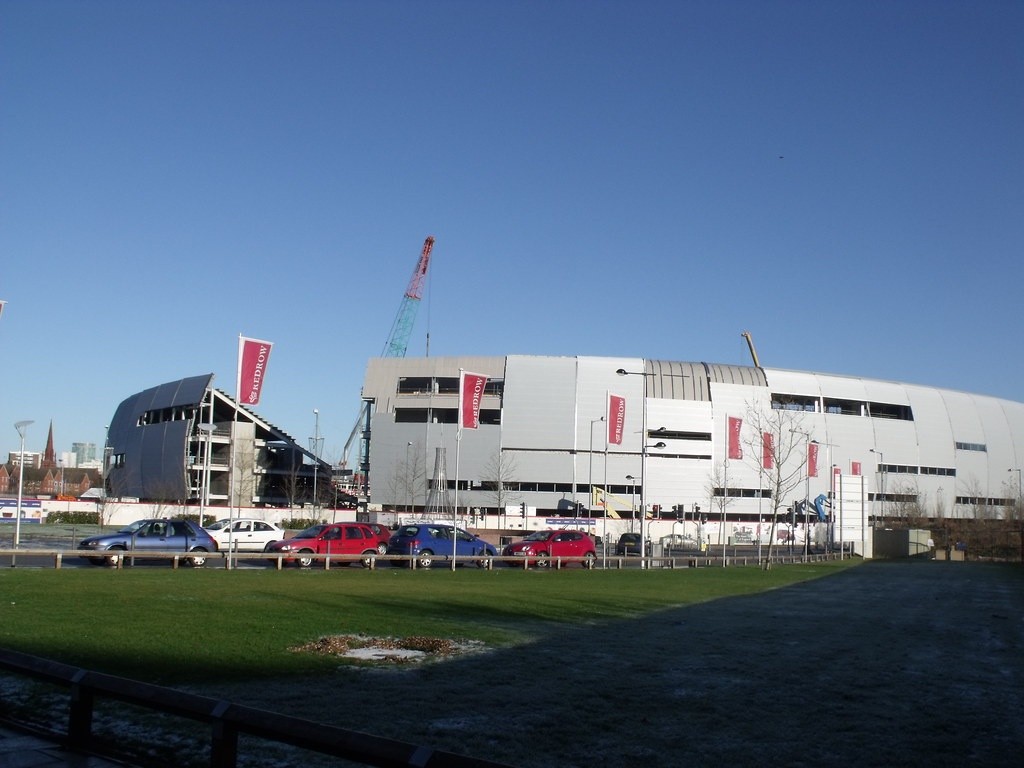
[202, 518, 285, 552]
[502, 529, 596, 568]
[387, 524, 498, 569]
[658, 535, 697, 550]
[75, 517, 219, 569]
[265, 524, 381, 568]
[338, 522, 392, 556]
[616, 532, 651, 555]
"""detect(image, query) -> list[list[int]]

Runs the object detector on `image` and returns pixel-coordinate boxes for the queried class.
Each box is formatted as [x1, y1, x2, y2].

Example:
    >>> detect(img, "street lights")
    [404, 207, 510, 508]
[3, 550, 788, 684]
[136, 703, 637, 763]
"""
[589, 417, 607, 537]
[58, 458, 64, 497]
[333, 481, 338, 524]
[617, 369, 666, 571]
[15, 419, 35, 552]
[830, 464, 836, 522]
[103, 425, 115, 498]
[870, 449, 883, 528]
[309, 408, 325, 521]
[404, 441, 412, 513]
[625, 474, 636, 534]
[810, 440, 833, 553]
[788, 429, 808, 564]
[1008, 468, 1021, 520]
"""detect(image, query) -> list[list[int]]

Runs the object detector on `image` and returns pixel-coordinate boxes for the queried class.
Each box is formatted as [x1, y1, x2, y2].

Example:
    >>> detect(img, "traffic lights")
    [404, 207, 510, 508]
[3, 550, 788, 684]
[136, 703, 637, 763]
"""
[652, 505, 659, 520]
[678, 504, 684, 519]
[480, 509, 484, 521]
[520, 503, 525, 519]
